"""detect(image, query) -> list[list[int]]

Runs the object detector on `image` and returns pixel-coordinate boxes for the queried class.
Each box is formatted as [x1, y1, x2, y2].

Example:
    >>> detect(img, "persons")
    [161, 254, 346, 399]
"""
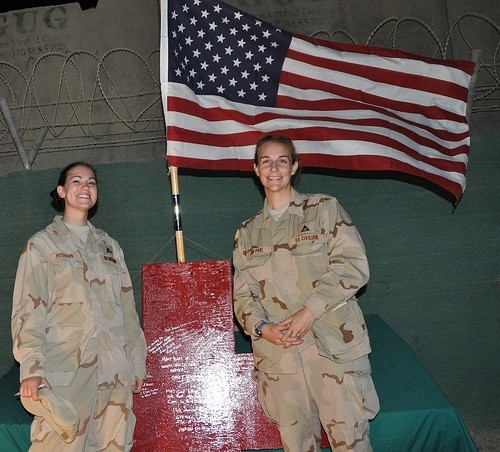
[11, 162, 147, 452]
[233, 135, 380, 452]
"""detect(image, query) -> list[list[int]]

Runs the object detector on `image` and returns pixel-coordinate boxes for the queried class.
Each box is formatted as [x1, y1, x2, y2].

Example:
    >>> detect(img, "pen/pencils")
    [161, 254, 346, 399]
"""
[14, 384, 45, 396]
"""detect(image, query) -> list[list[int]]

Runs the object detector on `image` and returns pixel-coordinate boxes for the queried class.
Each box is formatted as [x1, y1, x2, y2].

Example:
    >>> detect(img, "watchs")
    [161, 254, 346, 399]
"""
[256, 321, 267, 336]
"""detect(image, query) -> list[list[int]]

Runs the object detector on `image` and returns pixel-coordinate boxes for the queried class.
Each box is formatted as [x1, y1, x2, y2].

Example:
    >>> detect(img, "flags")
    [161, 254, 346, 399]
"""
[159, 0, 476, 205]
